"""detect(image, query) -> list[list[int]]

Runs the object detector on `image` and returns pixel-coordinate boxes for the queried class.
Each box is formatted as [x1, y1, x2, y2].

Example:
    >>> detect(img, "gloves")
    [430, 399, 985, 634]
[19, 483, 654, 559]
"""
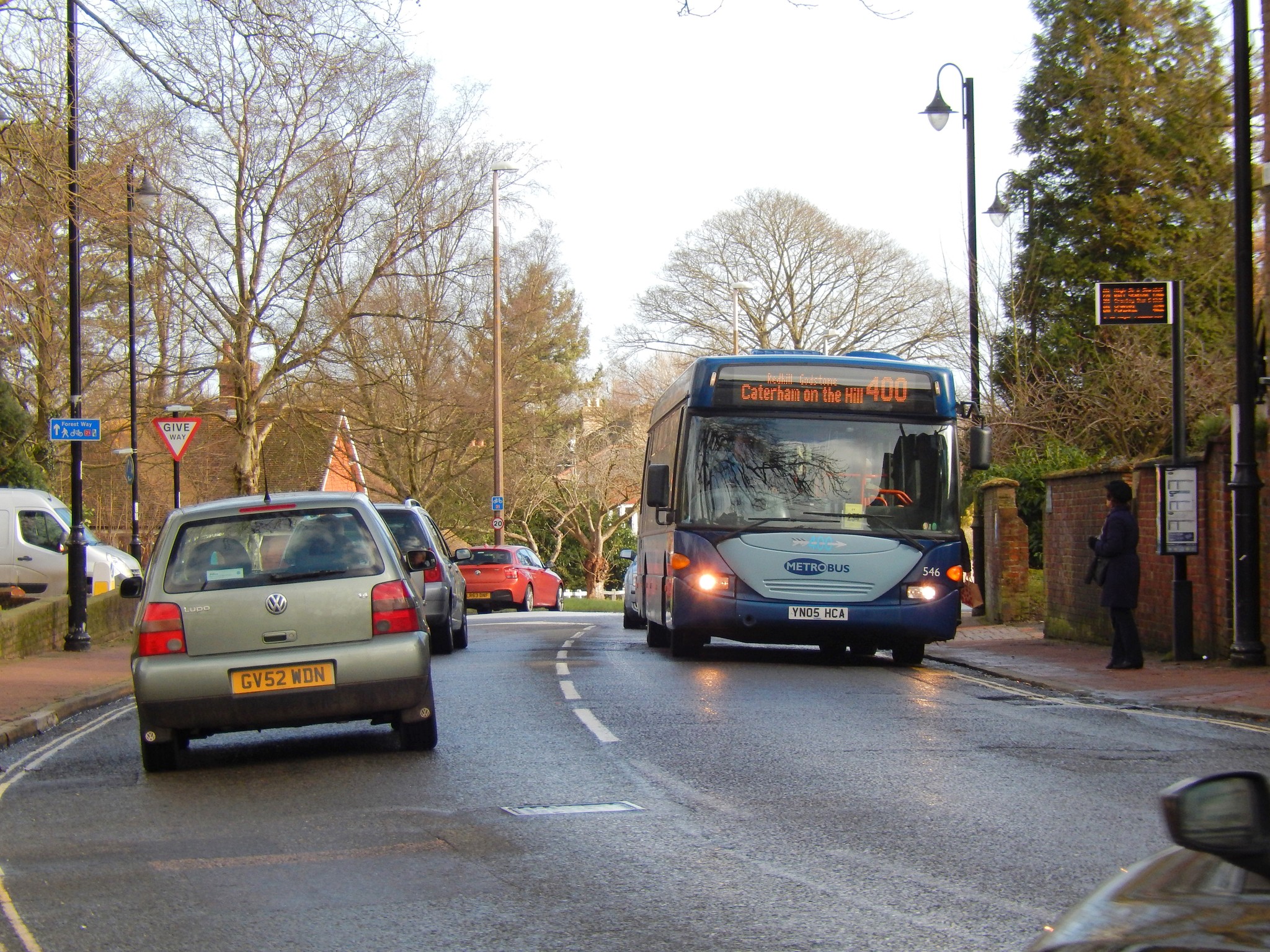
[1088, 537, 1099, 549]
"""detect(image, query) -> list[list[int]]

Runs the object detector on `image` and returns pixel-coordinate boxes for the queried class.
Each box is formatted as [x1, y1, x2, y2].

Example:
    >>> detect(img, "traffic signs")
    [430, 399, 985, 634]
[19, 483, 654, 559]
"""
[50, 419, 103, 442]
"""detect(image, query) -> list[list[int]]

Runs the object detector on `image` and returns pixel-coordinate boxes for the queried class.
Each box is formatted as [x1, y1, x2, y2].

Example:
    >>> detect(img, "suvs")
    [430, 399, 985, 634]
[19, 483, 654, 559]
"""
[0, 487, 143, 602]
[274, 499, 470, 656]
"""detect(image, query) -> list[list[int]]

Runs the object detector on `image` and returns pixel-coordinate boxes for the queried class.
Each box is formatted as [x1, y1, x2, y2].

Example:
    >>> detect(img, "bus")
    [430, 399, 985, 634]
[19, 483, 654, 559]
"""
[636, 347, 993, 668]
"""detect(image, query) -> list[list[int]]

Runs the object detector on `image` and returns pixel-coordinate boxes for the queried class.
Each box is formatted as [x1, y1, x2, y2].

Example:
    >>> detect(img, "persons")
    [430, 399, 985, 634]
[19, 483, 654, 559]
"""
[709, 430, 779, 492]
[1085, 480, 1146, 670]
[19, 511, 54, 551]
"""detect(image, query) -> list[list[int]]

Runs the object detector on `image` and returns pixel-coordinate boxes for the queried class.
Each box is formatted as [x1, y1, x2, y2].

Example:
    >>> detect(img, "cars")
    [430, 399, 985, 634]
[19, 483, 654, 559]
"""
[619, 550, 647, 629]
[114, 489, 439, 773]
[451, 545, 565, 613]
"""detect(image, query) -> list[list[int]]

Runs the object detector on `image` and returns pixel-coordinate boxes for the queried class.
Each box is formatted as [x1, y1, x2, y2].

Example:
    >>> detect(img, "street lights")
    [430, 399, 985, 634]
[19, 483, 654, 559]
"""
[123, 162, 165, 569]
[919, 62, 980, 614]
[488, 163, 522, 541]
[823, 327, 838, 355]
[730, 280, 753, 356]
[982, 172, 1040, 349]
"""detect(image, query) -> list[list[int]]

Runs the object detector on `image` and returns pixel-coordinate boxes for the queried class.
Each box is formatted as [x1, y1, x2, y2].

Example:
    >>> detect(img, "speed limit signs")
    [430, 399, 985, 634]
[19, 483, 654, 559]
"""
[491, 517, 505, 531]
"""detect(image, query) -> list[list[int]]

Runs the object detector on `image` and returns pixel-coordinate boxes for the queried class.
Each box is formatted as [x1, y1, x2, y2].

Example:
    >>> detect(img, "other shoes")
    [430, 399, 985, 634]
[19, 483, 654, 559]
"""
[1113, 659, 1143, 671]
[1106, 658, 1124, 669]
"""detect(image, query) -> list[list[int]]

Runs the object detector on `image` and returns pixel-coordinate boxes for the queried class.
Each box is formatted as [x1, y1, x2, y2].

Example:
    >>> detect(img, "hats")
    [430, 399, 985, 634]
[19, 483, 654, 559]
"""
[1104, 480, 1133, 503]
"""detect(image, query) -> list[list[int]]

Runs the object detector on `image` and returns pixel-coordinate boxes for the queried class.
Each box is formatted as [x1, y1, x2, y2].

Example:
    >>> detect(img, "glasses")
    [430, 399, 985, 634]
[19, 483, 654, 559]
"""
[1106, 494, 1116, 500]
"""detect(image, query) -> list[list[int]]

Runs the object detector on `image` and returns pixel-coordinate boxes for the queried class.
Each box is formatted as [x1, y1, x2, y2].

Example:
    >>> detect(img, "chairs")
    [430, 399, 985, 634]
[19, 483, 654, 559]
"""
[186, 537, 252, 580]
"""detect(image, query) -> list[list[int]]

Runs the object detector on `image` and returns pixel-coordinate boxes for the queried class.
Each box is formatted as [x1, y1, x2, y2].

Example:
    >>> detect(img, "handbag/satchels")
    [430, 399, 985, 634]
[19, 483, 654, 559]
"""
[1085, 557, 1110, 586]
